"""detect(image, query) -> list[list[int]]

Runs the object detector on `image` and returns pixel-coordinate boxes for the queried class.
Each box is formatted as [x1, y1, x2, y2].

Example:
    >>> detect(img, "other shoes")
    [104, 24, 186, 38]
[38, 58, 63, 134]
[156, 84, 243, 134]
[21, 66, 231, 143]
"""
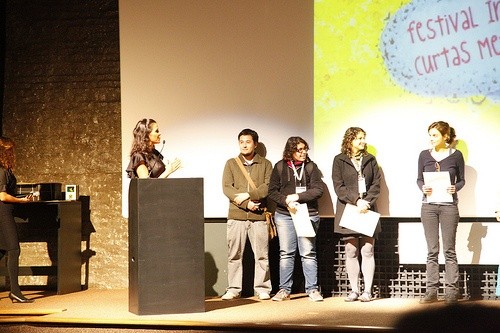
[221, 291, 239, 300]
[259, 292, 270, 300]
[444, 295, 455, 302]
[419, 292, 438, 303]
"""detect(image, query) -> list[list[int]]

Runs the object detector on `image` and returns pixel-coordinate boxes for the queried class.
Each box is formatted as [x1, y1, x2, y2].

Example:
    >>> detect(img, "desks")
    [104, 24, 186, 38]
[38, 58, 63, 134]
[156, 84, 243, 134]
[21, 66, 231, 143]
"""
[0, 199, 82, 295]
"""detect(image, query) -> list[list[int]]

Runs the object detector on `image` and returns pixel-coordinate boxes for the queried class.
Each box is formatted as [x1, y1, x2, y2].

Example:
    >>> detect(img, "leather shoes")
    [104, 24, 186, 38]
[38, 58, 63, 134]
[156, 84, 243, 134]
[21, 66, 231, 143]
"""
[344, 290, 358, 302]
[358, 292, 371, 302]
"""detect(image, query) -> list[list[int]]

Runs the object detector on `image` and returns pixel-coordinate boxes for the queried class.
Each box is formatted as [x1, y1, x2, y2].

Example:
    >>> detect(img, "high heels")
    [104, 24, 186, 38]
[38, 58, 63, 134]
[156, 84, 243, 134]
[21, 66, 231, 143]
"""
[9, 292, 29, 303]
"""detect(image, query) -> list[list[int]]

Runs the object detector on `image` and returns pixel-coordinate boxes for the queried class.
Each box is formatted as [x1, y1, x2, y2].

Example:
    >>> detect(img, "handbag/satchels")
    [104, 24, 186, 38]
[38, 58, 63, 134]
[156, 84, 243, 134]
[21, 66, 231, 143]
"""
[264, 211, 277, 239]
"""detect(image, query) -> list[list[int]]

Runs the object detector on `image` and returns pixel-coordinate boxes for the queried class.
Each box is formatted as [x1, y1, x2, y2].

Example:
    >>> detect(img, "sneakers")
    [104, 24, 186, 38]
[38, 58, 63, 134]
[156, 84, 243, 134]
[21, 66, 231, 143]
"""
[308, 290, 324, 301]
[272, 288, 291, 301]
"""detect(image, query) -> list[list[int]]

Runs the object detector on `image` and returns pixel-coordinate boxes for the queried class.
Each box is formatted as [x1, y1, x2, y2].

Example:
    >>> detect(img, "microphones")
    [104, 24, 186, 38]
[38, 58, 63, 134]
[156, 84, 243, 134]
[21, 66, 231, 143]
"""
[149, 140, 165, 174]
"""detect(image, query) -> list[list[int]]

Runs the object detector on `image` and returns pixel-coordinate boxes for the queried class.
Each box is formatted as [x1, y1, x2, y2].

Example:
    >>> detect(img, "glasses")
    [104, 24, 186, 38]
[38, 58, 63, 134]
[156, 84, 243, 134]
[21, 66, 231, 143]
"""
[296, 146, 308, 152]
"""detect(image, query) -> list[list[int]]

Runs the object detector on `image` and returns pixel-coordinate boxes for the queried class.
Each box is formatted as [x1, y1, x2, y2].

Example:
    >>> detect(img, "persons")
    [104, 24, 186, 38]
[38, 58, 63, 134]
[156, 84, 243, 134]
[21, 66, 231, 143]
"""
[331, 127, 380, 302]
[125, 118, 181, 179]
[268, 136, 325, 301]
[220, 129, 273, 300]
[416, 120, 465, 304]
[0, 135, 35, 304]
